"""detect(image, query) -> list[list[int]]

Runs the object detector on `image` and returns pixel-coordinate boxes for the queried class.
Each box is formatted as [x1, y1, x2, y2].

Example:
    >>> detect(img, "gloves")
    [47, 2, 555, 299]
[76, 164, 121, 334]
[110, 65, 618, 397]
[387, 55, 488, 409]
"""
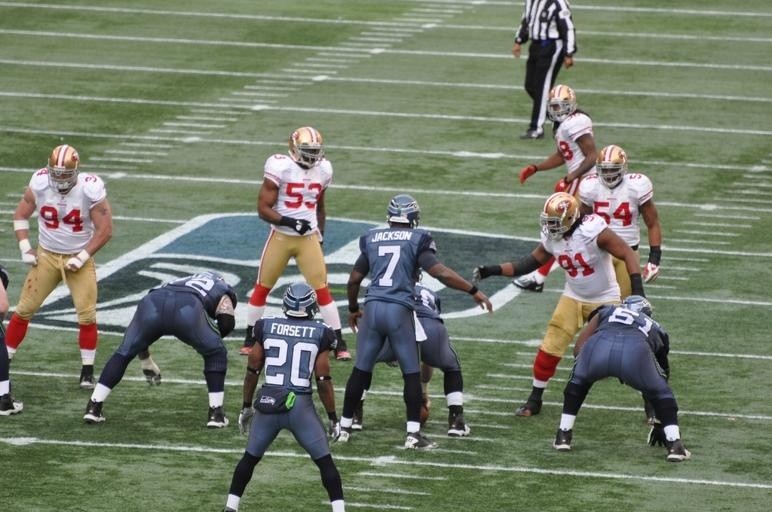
[238, 405, 254, 435]
[470, 264, 502, 285]
[327, 419, 341, 443]
[641, 252, 660, 286]
[646, 419, 668, 448]
[139, 355, 163, 386]
[517, 165, 538, 184]
[554, 175, 574, 197]
[276, 215, 312, 236]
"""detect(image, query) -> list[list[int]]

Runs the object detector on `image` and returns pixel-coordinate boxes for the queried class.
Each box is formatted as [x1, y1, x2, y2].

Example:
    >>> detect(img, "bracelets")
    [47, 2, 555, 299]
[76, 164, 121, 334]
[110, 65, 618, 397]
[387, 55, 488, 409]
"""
[11, 221, 31, 230]
[325, 412, 337, 422]
[19, 239, 31, 254]
[77, 249, 91, 263]
[468, 285, 478, 295]
[348, 306, 361, 314]
[242, 401, 252, 408]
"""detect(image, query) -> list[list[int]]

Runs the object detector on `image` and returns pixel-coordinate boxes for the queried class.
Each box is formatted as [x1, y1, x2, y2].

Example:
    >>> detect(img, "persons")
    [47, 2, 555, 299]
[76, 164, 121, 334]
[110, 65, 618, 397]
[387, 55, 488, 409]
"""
[333, 194, 492, 448]
[241, 126, 350, 362]
[512, 0, 577, 140]
[554, 294, 692, 462]
[512, 83, 598, 292]
[225, 282, 346, 512]
[578, 145, 661, 283]
[6, 145, 112, 390]
[82, 276, 237, 428]
[0, 266, 23, 416]
[352, 283, 470, 437]
[472, 192, 645, 416]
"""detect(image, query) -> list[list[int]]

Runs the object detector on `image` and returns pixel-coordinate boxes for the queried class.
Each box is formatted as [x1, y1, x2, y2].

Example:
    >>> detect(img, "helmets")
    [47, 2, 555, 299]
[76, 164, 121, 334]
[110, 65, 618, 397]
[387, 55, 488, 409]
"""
[547, 84, 577, 120]
[621, 293, 654, 319]
[281, 282, 319, 321]
[285, 125, 324, 163]
[386, 193, 422, 228]
[46, 144, 81, 174]
[543, 190, 581, 236]
[595, 143, 628, 183]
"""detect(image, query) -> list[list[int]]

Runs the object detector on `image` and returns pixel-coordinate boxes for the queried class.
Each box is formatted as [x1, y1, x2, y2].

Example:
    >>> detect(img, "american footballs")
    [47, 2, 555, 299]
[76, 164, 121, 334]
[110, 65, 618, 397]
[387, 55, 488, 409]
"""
[420, 404, 427, 423]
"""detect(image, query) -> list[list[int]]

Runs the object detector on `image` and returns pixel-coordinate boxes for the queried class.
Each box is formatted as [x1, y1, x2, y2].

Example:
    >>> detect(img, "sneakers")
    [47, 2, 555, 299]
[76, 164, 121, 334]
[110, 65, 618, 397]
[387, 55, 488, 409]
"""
[402, 432, 441, 452]
[515, 397, 545, 417]
[78, 374, 97, 389]
[347, 411, 365, 432]
[665, 433, 693, 462]
[520, 127, 545, 141]
[82, 400, 107, 424]
[0, 400, 24, 417]
[445, 415, 474, 437]
[334, 340, 352, 361]
[337, 426, 351, 443]
[553, 429, 575, 450]
[237, 341, 260, 354]
[206, 405, 231, 428]
[512, 274, 547, 292]
